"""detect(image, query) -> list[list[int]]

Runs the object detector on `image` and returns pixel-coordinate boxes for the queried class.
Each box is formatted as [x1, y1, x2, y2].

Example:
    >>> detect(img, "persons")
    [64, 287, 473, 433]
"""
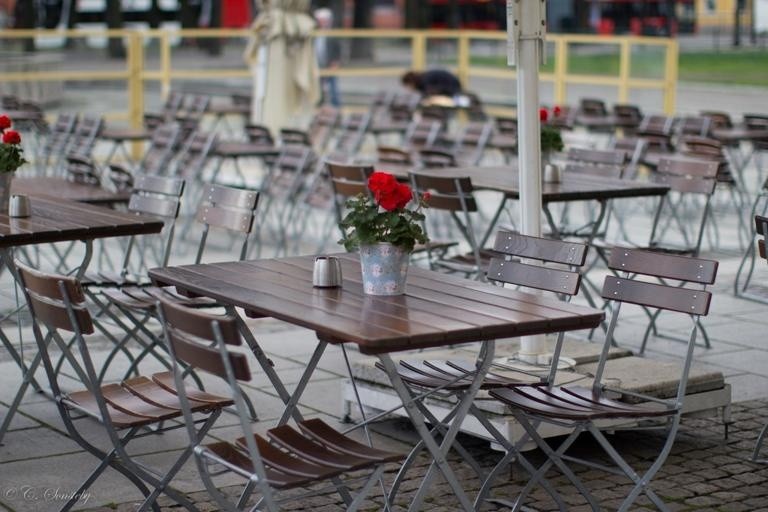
[311, 5, 341, 106]
